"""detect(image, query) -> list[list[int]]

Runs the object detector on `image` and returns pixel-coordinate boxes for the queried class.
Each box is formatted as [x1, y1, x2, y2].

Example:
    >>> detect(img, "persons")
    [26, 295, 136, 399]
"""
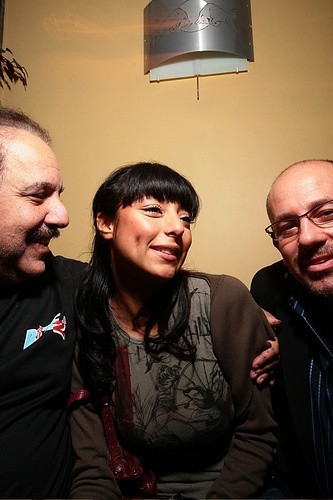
[251, 158, 333, 500]
[64, 157, 282, 500]
[1, 104, 297, 500]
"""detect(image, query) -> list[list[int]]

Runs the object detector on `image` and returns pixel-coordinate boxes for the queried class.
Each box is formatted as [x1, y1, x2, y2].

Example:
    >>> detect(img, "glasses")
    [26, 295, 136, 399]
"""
[264, 197, 333, 239]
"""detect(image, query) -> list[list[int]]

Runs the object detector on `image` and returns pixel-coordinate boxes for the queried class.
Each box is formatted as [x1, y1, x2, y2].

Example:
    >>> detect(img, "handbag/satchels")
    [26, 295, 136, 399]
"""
[69, 380, 160, 498]
